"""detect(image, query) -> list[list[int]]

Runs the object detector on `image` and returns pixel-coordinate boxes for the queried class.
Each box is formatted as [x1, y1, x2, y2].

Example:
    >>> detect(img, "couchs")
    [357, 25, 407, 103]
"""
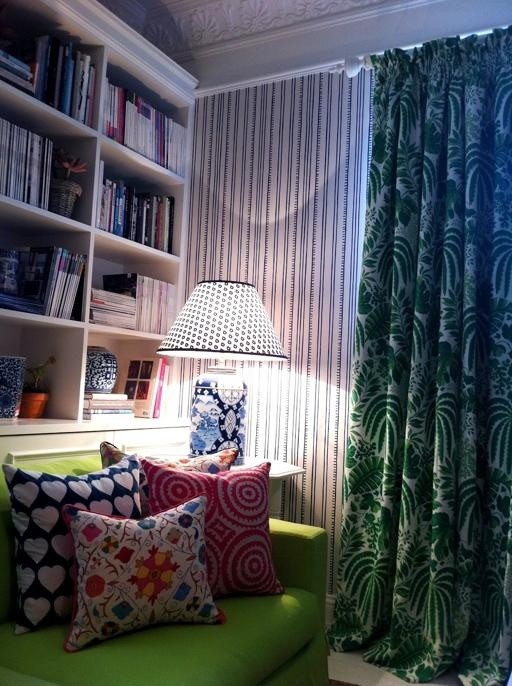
[1, 457, 331, 683]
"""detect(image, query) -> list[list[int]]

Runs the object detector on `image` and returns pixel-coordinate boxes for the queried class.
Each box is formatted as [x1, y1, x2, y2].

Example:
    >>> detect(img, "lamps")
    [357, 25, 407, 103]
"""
[154, 280, 289, 469]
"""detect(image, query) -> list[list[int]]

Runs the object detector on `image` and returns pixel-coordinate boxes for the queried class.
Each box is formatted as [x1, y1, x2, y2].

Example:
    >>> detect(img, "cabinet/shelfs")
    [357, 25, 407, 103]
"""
[0, 2, 203, 465]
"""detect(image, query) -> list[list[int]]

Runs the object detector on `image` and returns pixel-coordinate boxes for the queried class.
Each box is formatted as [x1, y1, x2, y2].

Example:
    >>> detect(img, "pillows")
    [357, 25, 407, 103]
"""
[3, 441, 283, 652]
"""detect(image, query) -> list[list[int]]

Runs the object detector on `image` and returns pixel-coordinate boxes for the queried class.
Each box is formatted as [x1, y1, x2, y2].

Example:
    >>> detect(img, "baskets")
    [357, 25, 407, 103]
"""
[48, 168, 83, 219]
